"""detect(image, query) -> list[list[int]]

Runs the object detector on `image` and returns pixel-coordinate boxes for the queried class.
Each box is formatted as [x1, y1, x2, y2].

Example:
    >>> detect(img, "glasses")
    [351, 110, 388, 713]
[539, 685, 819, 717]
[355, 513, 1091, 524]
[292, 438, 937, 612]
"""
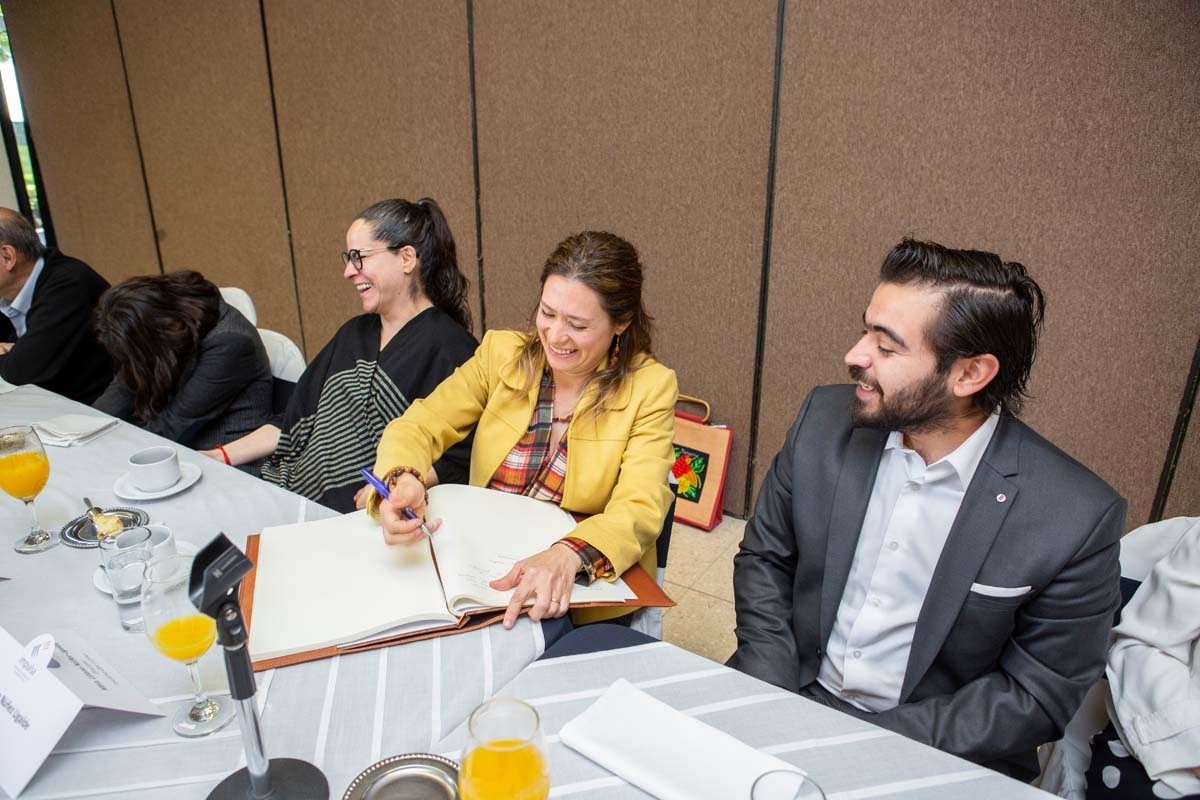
[342, 243, 419, 269]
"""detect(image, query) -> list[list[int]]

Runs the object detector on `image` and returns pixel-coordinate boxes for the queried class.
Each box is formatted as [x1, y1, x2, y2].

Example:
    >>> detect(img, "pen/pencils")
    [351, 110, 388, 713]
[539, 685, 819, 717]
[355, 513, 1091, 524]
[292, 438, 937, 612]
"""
[361, 469, 433, 536]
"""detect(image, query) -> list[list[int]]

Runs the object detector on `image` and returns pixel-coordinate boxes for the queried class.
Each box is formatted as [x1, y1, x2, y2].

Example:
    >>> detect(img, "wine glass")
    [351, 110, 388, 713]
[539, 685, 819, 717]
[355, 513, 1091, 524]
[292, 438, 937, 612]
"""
[0, 425, 60, 554]
[140, 554, 237, 738]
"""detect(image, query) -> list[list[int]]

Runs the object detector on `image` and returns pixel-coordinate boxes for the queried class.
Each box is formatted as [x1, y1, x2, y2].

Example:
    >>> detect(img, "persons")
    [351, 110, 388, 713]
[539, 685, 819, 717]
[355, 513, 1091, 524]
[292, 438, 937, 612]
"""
[89, 270, 272, 479]
[1086, 519, 1200, 800]
[196, 197, 480, 516]
[0, 207, 121, 407]
[726, 239, 1128, 786]
[365, 230, 680, 631]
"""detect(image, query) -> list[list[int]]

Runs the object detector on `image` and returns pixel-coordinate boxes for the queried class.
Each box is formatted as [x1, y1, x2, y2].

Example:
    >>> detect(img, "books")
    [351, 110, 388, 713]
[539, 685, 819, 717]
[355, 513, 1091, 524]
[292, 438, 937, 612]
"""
[239, 484, 678, 672]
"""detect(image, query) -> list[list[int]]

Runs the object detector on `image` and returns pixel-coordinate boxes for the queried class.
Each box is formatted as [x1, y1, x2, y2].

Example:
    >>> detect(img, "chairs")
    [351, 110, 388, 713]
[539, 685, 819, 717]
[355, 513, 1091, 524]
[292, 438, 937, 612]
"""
[219, 287, 306, 416]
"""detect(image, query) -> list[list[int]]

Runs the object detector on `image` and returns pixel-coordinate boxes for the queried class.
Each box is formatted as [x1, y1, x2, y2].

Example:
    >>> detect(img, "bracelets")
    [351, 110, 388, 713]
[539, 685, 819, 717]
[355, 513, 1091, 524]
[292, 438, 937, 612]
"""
[378, 468, 429, 506]
[215, 445, 231, 465]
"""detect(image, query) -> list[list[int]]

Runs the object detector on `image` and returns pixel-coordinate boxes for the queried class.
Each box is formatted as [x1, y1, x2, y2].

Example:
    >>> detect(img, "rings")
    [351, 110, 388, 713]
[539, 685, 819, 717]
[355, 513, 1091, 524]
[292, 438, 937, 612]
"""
[551, 599, 560, 605]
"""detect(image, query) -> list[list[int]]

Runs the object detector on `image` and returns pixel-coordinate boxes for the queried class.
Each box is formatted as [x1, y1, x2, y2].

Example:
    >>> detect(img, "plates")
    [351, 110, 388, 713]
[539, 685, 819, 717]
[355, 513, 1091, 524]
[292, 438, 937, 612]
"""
[112, 462, 202, 501]
[93, 542, 198, 595]
[59, 507, 149, 548]
[342, 753, 459, 800]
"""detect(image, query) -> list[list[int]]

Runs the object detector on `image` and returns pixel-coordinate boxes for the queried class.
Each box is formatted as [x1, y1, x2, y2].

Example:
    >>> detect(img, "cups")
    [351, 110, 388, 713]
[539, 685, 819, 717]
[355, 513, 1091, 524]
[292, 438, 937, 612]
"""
[127, 446, 181, 493]
[98, 523, 178, 632]
[458, 696, 551, 800]
[749, 769, 827, 800]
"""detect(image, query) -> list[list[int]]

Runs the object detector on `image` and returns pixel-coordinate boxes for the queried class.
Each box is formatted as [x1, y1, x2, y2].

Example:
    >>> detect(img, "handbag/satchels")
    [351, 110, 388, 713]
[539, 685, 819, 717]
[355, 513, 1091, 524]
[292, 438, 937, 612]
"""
[673, 394, 733, 531]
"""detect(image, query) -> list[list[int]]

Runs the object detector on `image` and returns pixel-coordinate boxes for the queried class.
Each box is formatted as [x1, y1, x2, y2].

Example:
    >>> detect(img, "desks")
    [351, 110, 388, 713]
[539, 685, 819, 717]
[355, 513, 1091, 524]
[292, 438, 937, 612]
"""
[0, 383, 1068, 800]
